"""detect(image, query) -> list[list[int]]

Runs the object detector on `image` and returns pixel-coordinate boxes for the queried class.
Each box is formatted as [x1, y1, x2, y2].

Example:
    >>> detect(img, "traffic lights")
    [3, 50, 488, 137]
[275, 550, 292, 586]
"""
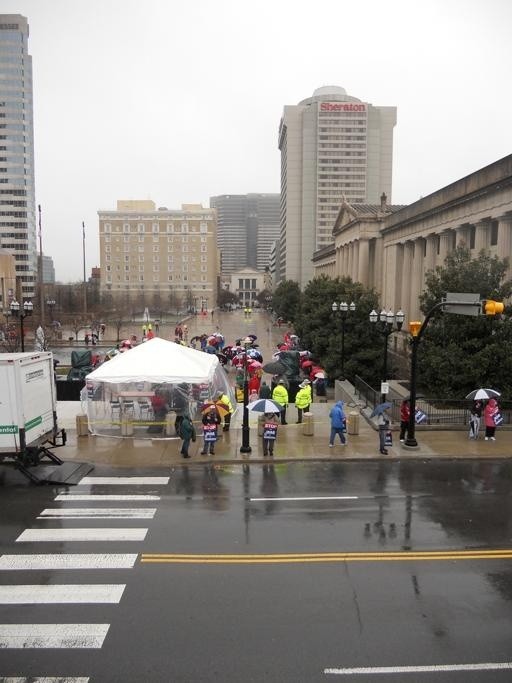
[485, 301, 503, 316]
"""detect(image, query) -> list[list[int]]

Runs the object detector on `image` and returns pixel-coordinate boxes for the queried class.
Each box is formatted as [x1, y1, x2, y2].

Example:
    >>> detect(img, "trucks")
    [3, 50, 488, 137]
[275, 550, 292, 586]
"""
[0, 351, 66, 466]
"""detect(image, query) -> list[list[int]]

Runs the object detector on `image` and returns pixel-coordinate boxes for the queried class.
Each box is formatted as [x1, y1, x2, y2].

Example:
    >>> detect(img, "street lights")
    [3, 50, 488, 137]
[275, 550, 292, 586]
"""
[240, 336, 252, 453]
[332, 301, 356, 381]
[192, 296, 198, 314]
[370, 307, 404, 404]
[46, 301, 55, 321]
[265, 296, 272, 308]
[10, 298, 33, 352]
[2, 311, 12, 328]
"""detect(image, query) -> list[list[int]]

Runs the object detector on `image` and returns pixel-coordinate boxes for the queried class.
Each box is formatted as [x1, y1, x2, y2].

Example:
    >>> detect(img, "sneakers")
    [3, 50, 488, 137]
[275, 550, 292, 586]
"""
[181, 451, 190, 458]
[201, 452, 214, 455]
[484, 436, 496, 441]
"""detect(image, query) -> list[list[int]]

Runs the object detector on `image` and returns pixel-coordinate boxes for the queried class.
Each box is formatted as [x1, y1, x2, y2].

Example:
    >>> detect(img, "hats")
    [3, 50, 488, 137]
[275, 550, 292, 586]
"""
[299, 378, 311, 389]
[278, 379, 285, 384]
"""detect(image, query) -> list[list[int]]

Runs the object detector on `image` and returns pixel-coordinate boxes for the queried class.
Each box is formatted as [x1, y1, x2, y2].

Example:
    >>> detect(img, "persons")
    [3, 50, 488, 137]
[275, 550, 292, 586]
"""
[378, 409, 388, 455]
[399, 399, 412, 442]
[329, 401, 348, 448]
[262, 407, 279, 456]
[179, 413, 193, 458]
[469, 399, 483, 441]
[83, 322, 329, 432]
[484, 399, 499, 441]
[200, 404, 221, 456]
[187, 301, 274, 318]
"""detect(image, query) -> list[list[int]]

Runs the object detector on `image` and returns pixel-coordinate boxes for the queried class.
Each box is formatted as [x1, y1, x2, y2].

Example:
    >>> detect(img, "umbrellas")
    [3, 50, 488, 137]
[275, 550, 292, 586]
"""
[465, 388, 502, 401]
[402, 394, 424, 401]
[369, 402, 392, 419]
